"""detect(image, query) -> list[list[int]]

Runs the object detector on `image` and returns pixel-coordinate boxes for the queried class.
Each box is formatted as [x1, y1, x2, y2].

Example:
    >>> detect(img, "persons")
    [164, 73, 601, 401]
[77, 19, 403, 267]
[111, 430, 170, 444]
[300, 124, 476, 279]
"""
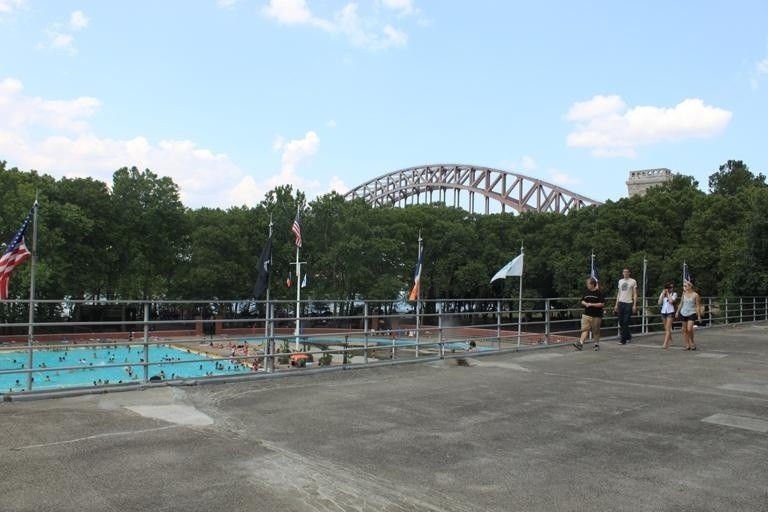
[675, 279, 704, 351]
[612, 267, 638, 345]
[466, 341, 479, 352]
[572, 275, 606, 353]
[657, 280, 678, 349]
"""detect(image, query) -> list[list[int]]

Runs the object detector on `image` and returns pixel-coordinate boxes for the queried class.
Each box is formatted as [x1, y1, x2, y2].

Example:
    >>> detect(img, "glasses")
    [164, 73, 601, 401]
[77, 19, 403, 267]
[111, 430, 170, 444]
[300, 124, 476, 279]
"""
[665, 286, 673, 289]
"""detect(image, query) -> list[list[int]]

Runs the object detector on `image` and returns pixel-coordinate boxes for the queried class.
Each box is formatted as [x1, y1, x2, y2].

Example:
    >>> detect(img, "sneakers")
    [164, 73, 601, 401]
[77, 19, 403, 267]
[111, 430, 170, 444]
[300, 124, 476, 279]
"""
[592, 342, 600, 351]
[618, 335, 631, 344]
[572, 341, 583, 350]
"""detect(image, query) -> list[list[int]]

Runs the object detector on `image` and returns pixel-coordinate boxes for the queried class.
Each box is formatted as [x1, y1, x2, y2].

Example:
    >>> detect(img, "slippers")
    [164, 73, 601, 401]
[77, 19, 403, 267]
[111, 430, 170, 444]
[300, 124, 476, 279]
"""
[686, 344, 696, 350]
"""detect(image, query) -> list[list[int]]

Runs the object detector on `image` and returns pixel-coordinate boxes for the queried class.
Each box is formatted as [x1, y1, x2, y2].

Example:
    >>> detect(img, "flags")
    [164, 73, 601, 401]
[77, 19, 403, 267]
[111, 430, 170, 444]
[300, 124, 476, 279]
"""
[408, 241, 423, 301]
[301, 273, 307, 288]
[683, 264, 695, 282]
[488, 252, 525, 284]
[252, 231, 273, 299]
[286, 272, 293, 288]
[0, 202, 34, 301]
[592, 255, 600, 283]
[291, 211, 303, 248]
